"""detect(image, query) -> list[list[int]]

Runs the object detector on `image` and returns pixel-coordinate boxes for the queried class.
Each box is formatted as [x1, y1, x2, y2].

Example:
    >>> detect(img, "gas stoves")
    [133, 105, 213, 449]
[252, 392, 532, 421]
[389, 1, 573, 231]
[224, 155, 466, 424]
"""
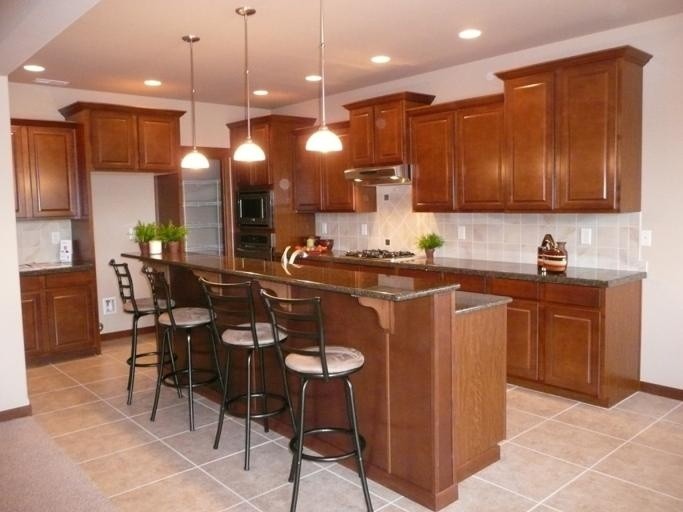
[346, 248, 415, 261]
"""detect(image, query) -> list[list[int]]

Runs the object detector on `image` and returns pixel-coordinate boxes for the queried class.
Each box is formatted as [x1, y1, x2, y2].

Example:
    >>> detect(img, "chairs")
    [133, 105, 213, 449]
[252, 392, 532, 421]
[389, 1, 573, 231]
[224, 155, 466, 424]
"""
[108, 259, 184, 406]
[139, 265, 228, 431]
[259, 287, 376, 512]
[196, 277, 295, 472]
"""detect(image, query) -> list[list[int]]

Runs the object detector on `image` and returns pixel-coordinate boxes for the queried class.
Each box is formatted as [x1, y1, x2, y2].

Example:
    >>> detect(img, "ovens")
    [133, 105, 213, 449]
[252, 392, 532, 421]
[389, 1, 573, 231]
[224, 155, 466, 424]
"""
[236, 183, 274, 261]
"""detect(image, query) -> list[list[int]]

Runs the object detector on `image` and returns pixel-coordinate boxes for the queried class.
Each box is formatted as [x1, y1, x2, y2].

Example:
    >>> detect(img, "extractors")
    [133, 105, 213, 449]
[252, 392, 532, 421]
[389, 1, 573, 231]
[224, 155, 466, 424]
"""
[342, 163, 411, 187]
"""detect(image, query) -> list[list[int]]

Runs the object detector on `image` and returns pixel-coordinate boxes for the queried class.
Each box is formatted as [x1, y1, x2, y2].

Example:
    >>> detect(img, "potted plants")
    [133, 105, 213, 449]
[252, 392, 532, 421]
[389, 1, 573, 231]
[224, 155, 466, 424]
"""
[159, 219, 188, 255]
[413, 230, 445, 258]
[132, 219, 159, 255]
[148, 221, 166, 254]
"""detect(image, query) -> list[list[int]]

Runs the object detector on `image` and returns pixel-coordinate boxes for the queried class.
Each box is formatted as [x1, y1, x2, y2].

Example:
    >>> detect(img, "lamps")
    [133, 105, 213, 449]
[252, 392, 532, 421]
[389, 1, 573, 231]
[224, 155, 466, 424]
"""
[306, 0, 343, 152]
[233, 7, 265, 162]
[180, 34, 210, 170]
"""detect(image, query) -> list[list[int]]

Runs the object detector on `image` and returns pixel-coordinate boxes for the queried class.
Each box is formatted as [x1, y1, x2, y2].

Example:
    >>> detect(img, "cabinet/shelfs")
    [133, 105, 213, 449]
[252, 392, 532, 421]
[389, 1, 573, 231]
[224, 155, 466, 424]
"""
[399, 268, 485, 296]
[340, 91, 436, 166]
[293, 118, 376, 214]
[494, 45, 654, 213]
[483, 280, 643, 410]
[323, 261, 398, 274]
[226, 113, 316, 257]
[10, 118, 83, 222]
[18, 261, 101, 370]
[404, 93, 502, 213]
[274, 254, 323, 267]
[56, 101, 187, 174]
